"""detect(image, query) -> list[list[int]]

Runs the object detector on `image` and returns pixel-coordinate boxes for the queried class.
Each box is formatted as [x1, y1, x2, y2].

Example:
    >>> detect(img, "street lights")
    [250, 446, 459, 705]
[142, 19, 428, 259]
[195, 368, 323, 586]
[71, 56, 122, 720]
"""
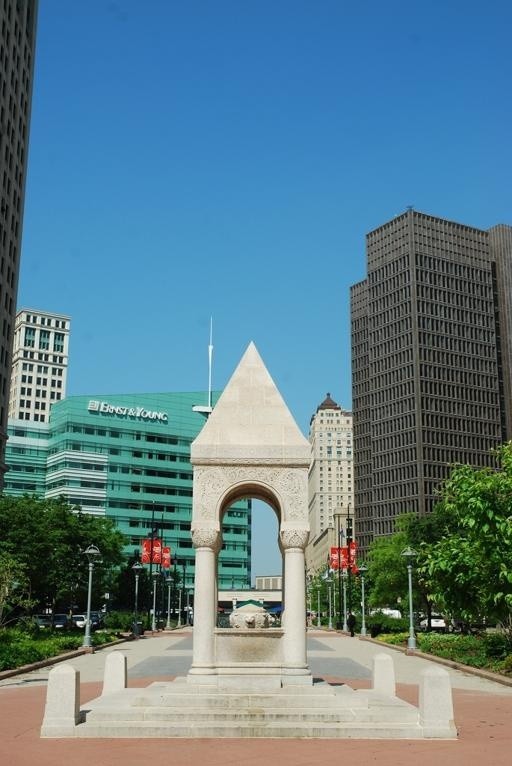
[401, 546, 419, 649]
[131, 561, 143, 627]
[358, 566, 368, 634]
[151, 571, 160, 631]
[326, 577, 336, 629]
[165, 575, 174, 628]
[316, 584, 324, 628]
[341, 572, 349, 633]
[177, 580, 184, 628]
[308, 584, 314, 626]
[82, 545, 102, 647]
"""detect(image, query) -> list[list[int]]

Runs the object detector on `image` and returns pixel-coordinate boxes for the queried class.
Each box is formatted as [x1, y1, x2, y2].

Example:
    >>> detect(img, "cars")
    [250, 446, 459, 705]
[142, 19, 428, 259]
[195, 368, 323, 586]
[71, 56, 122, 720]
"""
[71, 614, 87, 630]
[405, 610, 454, 634]
[51, 614, 69, 631]
[83, 610, 104, 631]
[31, 614, 51, 630]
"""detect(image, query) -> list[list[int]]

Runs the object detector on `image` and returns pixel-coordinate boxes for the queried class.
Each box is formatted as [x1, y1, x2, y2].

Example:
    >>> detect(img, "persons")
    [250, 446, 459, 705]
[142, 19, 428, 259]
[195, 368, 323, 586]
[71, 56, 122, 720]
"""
[347, 612, 356, 637]
[449, 622, 470, 634]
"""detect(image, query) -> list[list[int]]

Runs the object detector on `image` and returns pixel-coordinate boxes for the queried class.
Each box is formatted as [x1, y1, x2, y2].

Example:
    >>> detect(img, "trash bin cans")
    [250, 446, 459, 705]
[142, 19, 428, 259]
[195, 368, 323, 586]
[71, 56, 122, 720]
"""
[132, 622, 143, 635]
[155, 619, 164, 629]
